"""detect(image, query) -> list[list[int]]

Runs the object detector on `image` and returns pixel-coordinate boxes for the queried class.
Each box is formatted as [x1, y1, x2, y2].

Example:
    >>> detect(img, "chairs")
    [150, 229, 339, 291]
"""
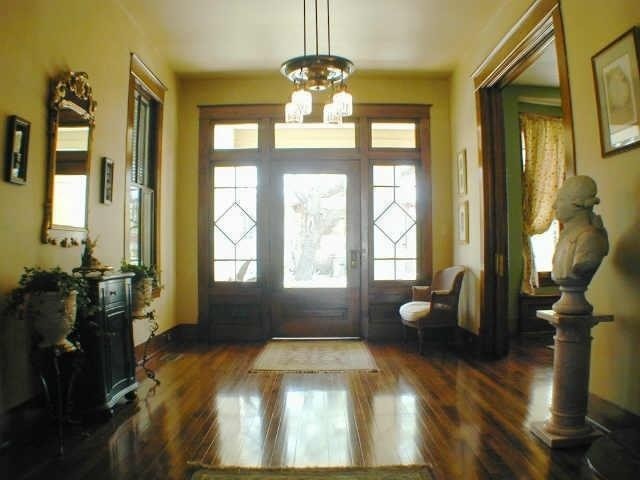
[398, 264, 466, 356]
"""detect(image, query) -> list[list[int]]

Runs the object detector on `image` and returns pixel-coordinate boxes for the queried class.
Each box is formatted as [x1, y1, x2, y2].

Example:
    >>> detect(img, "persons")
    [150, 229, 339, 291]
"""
[547, 174, 611, 288]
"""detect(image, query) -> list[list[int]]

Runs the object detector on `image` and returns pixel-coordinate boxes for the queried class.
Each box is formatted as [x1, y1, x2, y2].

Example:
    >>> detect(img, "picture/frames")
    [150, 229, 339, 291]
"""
[456, 147, 468, 248]
[590, 24, 638, 160]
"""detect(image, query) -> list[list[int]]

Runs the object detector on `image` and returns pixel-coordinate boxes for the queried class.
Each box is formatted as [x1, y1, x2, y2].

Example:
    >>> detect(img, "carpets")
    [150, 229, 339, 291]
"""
[183, 460, 438, 479]
[247, 337, 380, 375]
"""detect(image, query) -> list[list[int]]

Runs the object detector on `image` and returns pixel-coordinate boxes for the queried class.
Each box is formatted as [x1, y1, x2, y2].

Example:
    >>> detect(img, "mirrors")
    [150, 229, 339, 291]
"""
[42, 71, 99, 246]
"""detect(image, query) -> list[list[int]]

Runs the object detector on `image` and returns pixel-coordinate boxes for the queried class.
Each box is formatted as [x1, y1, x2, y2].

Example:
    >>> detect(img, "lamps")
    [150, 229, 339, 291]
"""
[276, 0, 357, 129]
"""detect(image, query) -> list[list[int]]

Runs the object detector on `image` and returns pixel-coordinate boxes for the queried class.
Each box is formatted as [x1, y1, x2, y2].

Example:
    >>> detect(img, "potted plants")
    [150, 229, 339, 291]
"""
[2, 265, 97, 353]
[117, 260, 164, 317]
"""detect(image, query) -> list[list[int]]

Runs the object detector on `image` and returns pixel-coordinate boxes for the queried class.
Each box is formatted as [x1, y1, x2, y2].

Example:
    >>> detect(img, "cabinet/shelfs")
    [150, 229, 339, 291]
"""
[74, 275, 138, 418]
